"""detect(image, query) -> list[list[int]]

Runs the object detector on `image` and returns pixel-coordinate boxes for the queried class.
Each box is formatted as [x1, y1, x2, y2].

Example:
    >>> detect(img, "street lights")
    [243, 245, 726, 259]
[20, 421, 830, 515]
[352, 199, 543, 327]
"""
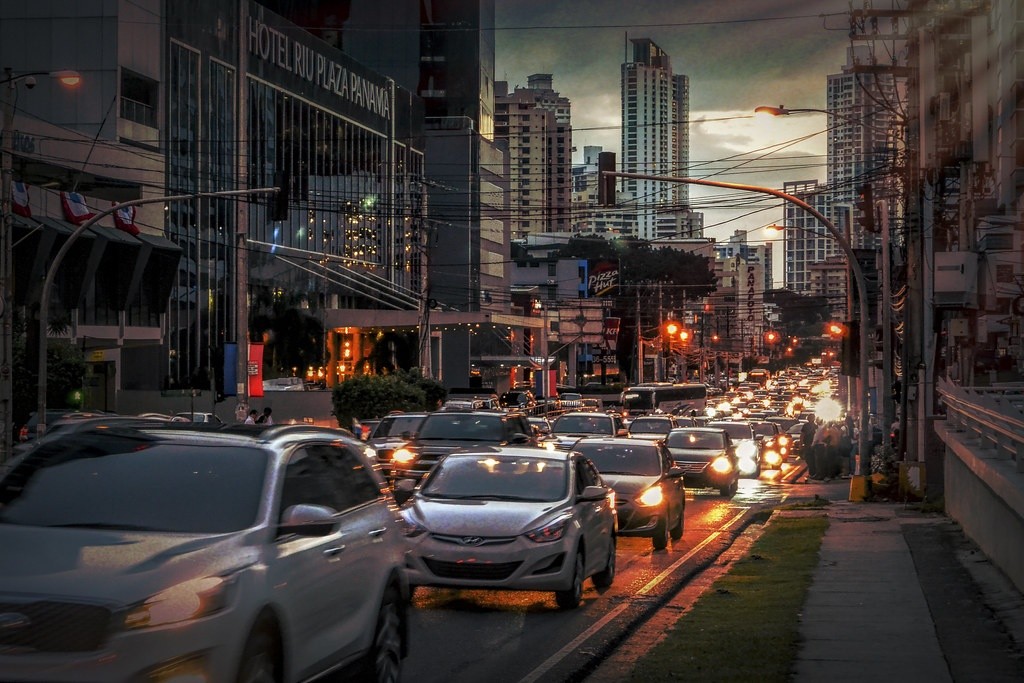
[756, 105, 922, 460]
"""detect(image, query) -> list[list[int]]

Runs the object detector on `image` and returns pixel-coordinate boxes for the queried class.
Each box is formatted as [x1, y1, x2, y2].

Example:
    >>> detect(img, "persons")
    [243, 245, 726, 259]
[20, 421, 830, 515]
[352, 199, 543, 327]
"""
[360, 423, 371, 443]
[244, 410, 258, 424]
[255, 407, 272, 424]
[799, 410, 900, 484]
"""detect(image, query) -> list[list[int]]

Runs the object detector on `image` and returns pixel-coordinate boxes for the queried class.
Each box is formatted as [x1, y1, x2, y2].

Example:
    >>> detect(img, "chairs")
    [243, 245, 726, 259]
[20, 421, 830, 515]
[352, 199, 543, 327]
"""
[538, 466, 564, 499]
[448, 464, 484, 496]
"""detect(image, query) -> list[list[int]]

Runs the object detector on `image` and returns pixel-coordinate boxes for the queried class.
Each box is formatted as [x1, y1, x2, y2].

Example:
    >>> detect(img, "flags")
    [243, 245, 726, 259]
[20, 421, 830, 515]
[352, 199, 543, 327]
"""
[60, 191, 96, 222]
[112, 200, 139, 234]
[11, 181, 31, 217]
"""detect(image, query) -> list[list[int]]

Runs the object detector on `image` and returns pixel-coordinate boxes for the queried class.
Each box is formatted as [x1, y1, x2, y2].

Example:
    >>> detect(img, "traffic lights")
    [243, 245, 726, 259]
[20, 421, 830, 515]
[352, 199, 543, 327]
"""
[854, 183, 874, 231]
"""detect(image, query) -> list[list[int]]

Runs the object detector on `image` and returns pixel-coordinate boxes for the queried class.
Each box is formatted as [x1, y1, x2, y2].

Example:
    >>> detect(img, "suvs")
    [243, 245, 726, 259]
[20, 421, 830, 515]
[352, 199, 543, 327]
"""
[541, 413, 628, 452]
[365, 411, 431, 483]
[627, 416, 678, 444]
[0, 421, 413, 683]
[21, 412, 108, 442]
[435, 391, 537, 415]
[395, 409, 538, 503]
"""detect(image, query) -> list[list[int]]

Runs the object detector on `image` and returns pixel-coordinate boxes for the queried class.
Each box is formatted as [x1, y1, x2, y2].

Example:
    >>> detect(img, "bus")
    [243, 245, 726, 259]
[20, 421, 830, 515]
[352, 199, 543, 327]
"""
[749, 369, 770, 389]
[620, 383, 707, 417]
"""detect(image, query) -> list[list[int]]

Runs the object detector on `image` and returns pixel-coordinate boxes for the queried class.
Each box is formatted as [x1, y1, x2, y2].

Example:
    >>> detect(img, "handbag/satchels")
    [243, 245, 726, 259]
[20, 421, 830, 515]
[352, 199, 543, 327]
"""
[840, 436, 853, 457]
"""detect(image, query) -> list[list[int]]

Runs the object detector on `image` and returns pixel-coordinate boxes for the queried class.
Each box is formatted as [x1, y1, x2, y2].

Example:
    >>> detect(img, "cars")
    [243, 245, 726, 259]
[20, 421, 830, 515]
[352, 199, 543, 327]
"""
[662, 426, 741, 497]
[706, 420, 765, 478]
[398, 447, 617, 608]
[772, 365, 838, 399]
[552, 438, 686, 549]
[744, 399, 835, 469]
[558, 392, 583, 412]
[176, 412, 223, 425]
[707, 384, 768, 422]
